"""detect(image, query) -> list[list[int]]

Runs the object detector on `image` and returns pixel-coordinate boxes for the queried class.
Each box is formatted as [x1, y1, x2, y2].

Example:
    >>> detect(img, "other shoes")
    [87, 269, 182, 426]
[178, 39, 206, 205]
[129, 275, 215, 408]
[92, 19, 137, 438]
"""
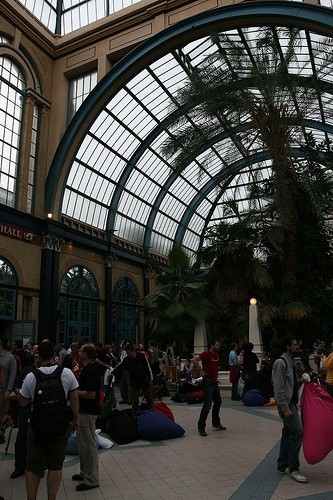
[289, 470, 308, 482]
[0, 416, 13, 432]
[72, 474, 84, 481]
[277, 467, 289, 474]
[10, 471, 23, 479]
[76, 482, 99, 491]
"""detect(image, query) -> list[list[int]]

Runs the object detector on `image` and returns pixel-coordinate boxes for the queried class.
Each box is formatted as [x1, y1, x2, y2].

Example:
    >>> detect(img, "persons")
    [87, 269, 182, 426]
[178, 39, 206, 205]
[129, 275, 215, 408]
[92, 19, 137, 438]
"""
[0, 338, 202, 500]
[193, 339, 227, 436]
[229, 343, 241, 400]
[322, 342, 333, 396]
[272, 335, 308, 483]
[243, 342, 260, 371]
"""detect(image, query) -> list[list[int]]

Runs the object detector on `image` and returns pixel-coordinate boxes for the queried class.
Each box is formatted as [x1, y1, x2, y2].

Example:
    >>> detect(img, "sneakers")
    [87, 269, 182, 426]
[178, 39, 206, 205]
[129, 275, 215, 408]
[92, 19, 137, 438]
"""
[212, 424, 226, 431]
[199, 427, 207, 436]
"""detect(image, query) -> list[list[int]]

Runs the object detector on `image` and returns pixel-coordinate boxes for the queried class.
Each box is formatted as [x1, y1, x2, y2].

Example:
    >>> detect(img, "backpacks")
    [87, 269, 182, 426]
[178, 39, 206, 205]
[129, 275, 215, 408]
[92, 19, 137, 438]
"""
[171, 382, 199, 403]
[31, 365, 74, 431]
[260, 357, 287, 398]
[180, 368, 187, 379]
[158, 386, 171, 401]
[149, 401, 174, 422]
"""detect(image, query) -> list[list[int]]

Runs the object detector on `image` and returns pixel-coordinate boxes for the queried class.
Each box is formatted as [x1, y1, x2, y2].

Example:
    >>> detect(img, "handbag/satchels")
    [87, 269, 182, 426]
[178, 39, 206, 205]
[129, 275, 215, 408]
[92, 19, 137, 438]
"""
[65, 433, 100, 455]
[136, 410, 185, 439]
[300, 381, 333, 465]
[95, 428, 114, 449]
[186, 370, 192, 383]
[96, 391, 154, 444]
[242, 370, 255, 382]
[242, 388, 266, 406]
[187, 390, 204, 404]
